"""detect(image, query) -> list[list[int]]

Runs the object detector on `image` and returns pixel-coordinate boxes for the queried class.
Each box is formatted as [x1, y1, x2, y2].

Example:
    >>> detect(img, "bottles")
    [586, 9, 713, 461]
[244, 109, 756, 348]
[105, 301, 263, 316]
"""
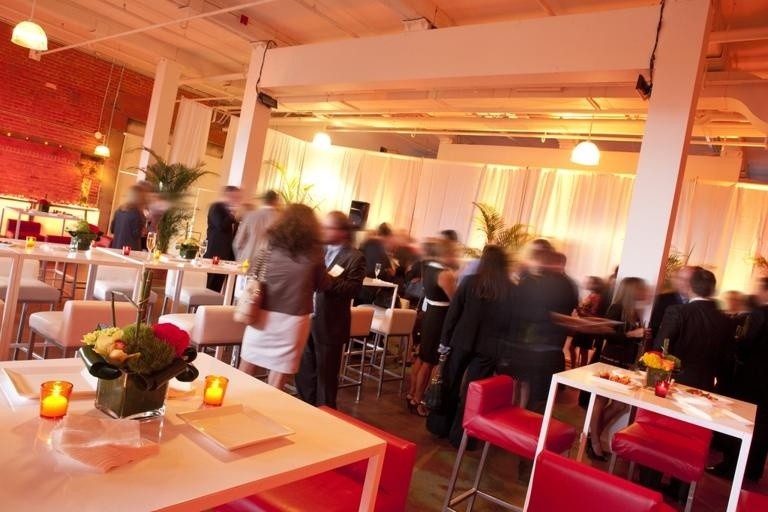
[42, 191, 49, 202]
[90, 240, 96, 255]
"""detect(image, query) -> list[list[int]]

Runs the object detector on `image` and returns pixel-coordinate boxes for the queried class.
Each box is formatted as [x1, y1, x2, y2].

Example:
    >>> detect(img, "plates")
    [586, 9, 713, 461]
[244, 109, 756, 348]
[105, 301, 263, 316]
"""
[1, 364, 101, 400]
[0, 239, 17, 247]
[673, 387, 755, 427]
[584, 371, 641, 390]
[173, 402, 296, 451]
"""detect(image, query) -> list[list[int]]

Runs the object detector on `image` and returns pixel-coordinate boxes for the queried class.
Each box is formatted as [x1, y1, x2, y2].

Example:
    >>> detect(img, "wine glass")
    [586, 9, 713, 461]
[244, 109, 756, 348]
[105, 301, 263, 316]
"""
[146, 232, 156, 260]
[374, 262, 382, 280]
[199, 239, 208, 263]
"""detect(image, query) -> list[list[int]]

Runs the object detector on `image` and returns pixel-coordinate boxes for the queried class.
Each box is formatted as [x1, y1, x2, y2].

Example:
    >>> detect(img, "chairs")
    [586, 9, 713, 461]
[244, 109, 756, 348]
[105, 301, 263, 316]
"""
[692, 456, 768, 512]
[526, 449, 680, 512]
[253, 388, 418, 512]
[0, 204, 417, 405]
[608, 407, 713, 512]
[444, 374, 578, 512]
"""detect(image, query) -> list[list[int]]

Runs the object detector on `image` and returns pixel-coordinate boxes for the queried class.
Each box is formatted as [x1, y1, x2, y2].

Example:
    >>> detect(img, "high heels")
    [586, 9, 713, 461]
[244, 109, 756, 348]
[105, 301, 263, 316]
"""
[405, 394, 427, 416]
[588, 436, 611, 461]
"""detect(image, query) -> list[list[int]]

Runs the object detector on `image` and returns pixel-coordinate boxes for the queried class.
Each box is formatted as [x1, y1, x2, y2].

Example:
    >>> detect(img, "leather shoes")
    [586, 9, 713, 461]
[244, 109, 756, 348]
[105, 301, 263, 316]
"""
[705, 465, 721, 475]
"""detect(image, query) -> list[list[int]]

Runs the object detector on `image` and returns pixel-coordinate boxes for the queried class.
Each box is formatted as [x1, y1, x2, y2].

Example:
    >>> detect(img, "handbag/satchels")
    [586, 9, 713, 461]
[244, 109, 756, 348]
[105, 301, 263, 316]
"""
[235, 277, 264, 323]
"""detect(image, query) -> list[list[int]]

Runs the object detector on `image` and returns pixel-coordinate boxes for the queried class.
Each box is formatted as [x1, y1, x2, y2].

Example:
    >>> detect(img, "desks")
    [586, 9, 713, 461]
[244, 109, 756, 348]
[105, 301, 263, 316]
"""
[523, 362, 758, 512]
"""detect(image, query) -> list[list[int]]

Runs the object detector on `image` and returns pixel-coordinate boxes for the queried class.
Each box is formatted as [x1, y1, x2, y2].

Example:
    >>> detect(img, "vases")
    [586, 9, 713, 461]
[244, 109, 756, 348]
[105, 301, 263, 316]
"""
[94, 358, 173, 420]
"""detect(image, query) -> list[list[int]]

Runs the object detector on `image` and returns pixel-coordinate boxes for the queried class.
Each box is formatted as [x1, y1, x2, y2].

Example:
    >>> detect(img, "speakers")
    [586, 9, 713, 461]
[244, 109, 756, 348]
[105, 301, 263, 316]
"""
[349, 202, 370, 227]
[259, 92, 278, 108]
[635, 73, 651, 99]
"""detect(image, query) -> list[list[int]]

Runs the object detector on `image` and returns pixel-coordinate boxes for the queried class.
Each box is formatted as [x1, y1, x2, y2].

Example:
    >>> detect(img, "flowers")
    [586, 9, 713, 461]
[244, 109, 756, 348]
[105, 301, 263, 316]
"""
[638, 335, 682, 370]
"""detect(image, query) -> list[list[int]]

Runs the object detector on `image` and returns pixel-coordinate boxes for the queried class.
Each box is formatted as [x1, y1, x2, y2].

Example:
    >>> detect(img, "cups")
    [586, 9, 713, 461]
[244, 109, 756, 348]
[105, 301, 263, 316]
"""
[202, 374, 229, 407]
[38, 380, 74, 418]
[26, 236, 36, 248]
[213, 255, 219, 265]
[70, 238, 78, 252]
[154, 250, 161, 260]
[654, 380, 670, 398]
[243, 258, 250, 267]
[123, 246, 131, 256]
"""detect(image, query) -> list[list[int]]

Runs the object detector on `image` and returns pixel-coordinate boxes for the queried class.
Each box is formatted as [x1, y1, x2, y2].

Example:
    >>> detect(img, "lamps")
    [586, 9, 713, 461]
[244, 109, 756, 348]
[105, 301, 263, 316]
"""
[309, 116, 333, 151]
[9, 0, 52, 52]
[570, 94, 602, 167]
[256, 91, 279, 109]
[635, 73, 653, 100]
[93, 57, 128, 158]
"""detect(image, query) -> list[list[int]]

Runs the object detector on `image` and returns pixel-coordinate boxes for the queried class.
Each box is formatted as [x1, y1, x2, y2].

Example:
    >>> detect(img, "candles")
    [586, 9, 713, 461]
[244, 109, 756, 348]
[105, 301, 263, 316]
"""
[40, 381, 69, 418]
